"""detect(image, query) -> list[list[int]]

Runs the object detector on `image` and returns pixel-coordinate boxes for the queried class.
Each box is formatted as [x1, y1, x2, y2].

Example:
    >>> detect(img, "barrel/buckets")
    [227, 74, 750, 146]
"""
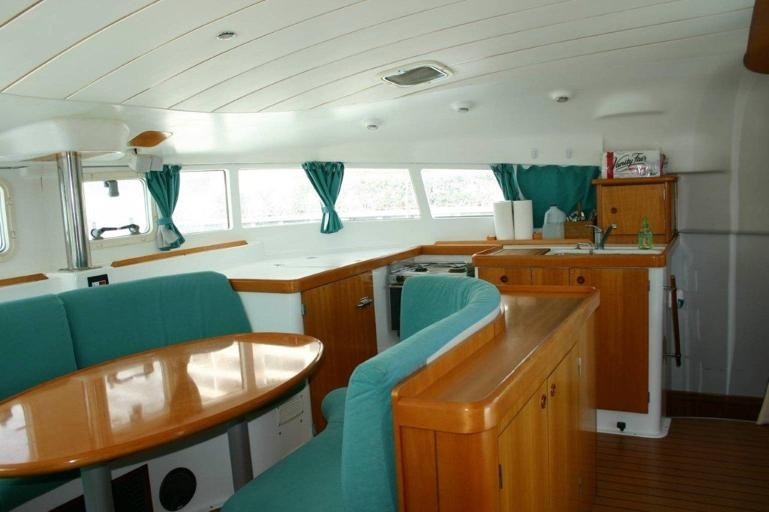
[542, 204, 566, 240]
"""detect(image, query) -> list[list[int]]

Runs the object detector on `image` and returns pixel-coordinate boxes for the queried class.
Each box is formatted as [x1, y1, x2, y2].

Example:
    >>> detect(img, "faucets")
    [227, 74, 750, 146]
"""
[584, 223, 618, 248]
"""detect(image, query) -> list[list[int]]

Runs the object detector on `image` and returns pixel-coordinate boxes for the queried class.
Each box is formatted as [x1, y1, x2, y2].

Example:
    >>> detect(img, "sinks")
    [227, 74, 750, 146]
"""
[553, 250, 592, 256]
[592, 246, 662, 254]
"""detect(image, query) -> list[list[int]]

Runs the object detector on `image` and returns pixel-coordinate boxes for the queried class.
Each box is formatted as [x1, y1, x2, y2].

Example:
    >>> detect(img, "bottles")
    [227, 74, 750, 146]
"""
[638, 216, 654, 249]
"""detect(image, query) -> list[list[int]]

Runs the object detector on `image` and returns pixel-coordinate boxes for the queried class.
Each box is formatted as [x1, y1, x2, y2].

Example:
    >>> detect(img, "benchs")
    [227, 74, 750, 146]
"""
[0, 268, 254, 512]
[219, 273, 500, 512]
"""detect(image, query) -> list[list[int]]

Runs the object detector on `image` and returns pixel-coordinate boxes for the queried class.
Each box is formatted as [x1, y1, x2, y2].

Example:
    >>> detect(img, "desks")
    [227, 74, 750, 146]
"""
[0, 331, 324, 512]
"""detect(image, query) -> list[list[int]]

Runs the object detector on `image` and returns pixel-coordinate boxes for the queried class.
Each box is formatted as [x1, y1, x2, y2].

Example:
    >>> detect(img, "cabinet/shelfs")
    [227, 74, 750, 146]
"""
[592, 175, 678, 245]
